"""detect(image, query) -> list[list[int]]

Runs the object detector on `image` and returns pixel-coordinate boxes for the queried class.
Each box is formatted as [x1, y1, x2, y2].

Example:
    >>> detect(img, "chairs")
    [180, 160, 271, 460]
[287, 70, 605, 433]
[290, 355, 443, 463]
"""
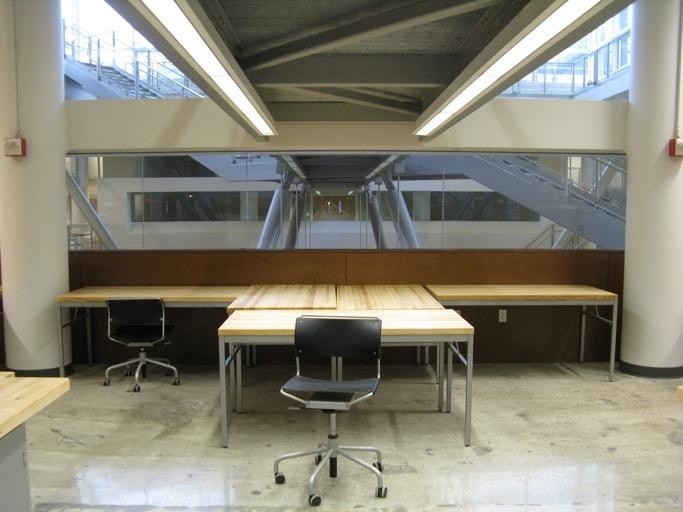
[103, 297, 179, 391]
[275, 315, 387, 506]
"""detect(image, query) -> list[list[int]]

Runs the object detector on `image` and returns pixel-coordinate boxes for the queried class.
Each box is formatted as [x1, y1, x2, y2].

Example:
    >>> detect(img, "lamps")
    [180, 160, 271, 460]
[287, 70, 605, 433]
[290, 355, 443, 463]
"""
[106, 0, 277, 143]
[410, 0, 641, 143]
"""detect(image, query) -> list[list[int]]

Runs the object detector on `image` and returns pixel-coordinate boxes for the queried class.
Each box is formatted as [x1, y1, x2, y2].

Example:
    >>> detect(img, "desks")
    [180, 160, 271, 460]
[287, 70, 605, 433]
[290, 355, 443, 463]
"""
[0, 375, 71, 512]
[424, 286, 619, 383]
[226, 287, 336, 408]
[337, 287, 445, 412]
[56, 286, 251, 377]
[217, 309, 476, 447]
